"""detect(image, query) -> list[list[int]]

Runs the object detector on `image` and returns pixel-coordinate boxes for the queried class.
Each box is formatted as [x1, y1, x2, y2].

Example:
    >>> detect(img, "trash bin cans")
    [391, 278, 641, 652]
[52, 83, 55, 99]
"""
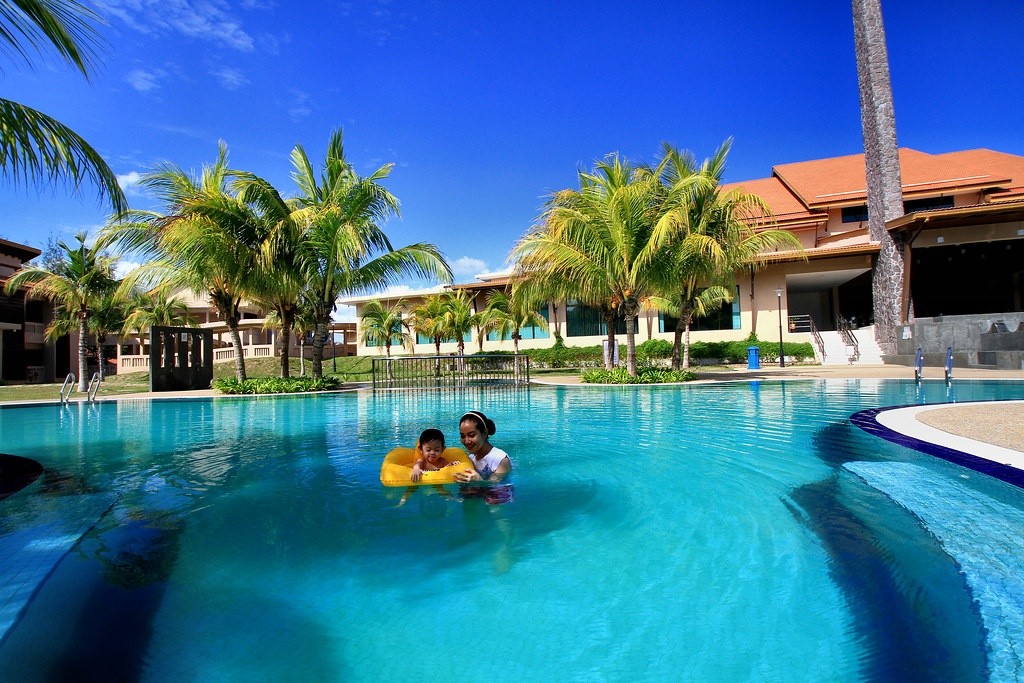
[747, 346, 761, 369]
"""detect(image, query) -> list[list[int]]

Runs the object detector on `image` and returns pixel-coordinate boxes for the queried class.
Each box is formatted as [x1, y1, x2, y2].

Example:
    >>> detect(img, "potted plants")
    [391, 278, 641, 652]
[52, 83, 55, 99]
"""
[789, 316, 799, 329]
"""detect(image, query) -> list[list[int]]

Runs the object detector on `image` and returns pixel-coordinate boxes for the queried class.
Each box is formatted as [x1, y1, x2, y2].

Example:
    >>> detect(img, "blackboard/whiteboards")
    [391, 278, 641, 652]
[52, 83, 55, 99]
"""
[603, 339, 619, 365]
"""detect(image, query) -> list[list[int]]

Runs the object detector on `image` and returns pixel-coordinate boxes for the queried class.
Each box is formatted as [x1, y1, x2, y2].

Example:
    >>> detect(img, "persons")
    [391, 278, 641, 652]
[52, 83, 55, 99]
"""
[410, 429, 460, 482]
[451, 411, 512, 483]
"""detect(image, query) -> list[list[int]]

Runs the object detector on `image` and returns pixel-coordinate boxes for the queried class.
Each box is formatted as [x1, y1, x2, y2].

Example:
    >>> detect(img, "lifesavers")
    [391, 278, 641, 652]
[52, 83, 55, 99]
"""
[380, 447, 475, 487]
